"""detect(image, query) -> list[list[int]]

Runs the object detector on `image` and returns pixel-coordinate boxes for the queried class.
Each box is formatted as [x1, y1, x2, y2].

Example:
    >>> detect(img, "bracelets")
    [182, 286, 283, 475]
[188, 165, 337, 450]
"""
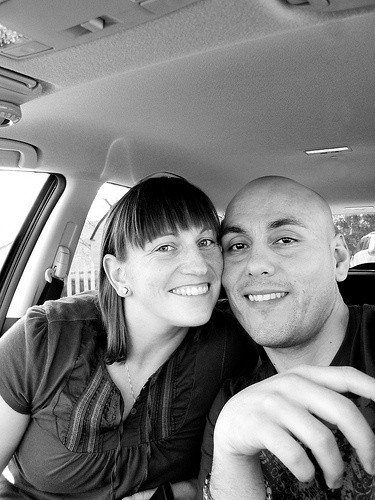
[203, 472, 273, 500]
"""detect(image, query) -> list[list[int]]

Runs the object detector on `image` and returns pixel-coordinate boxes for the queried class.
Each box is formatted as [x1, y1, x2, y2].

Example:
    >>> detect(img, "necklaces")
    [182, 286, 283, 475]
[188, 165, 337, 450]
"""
[125, 363, 137, 404]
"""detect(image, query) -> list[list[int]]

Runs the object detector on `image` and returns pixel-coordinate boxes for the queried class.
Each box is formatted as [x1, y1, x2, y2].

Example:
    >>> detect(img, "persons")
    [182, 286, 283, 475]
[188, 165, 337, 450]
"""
[0, 176, 250, 499]
[200, 175, 374, 499]
